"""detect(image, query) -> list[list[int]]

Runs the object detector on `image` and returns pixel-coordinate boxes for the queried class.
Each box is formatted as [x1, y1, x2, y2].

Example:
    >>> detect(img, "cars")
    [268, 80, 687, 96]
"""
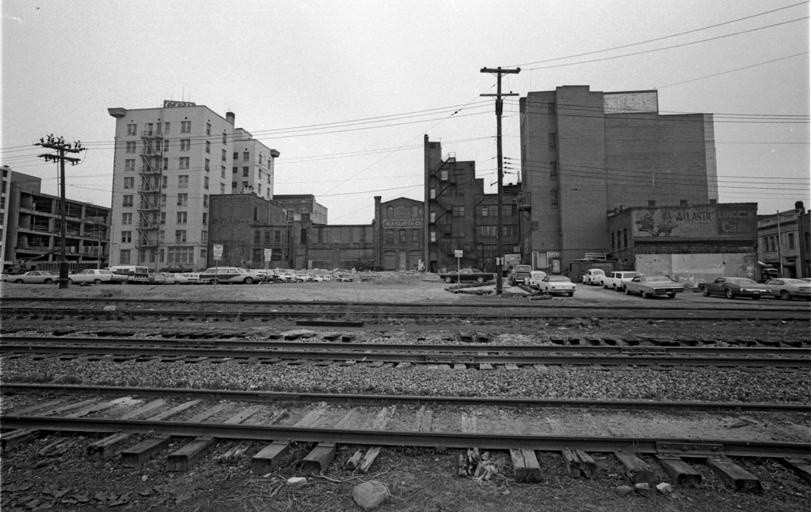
[624, 274, 685, 301]
[260, 267, 363, 284]
[583, 268, 607, 287]
[69, 268, 115, 284]
[199, 265, 265, 284]
[699, 276, 773, 300]
[154, 264, 200, 284]
[762, 278, 811, 303]
[4, 270, 61, 283]
[439, 262, 577, 296]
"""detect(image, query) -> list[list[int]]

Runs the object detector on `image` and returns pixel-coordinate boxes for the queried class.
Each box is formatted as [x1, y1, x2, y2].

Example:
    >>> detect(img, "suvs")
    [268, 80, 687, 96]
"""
[110, 266, 150, 284]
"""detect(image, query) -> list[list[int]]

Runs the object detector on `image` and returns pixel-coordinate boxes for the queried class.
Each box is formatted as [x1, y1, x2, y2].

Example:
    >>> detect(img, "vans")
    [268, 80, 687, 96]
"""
[602, 270, 642, 290]
